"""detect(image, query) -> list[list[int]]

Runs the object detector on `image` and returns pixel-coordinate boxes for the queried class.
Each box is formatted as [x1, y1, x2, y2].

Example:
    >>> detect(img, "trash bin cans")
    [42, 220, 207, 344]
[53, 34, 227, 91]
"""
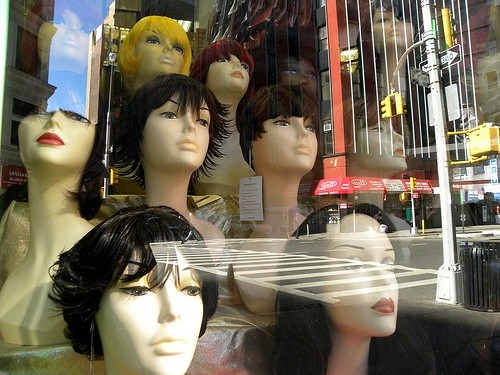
[459, 240, 500, 313]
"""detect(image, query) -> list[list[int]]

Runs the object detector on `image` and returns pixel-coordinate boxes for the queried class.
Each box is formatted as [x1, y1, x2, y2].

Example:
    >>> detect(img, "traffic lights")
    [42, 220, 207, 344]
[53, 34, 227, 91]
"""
[408, 176, 417, 191]
[441, 7, 455, 49]
[381, 92, 407, 119]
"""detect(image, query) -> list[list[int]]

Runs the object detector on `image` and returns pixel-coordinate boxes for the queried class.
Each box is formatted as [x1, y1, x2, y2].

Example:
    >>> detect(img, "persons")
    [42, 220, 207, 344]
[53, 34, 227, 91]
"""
[0, 0, 425, 375]
